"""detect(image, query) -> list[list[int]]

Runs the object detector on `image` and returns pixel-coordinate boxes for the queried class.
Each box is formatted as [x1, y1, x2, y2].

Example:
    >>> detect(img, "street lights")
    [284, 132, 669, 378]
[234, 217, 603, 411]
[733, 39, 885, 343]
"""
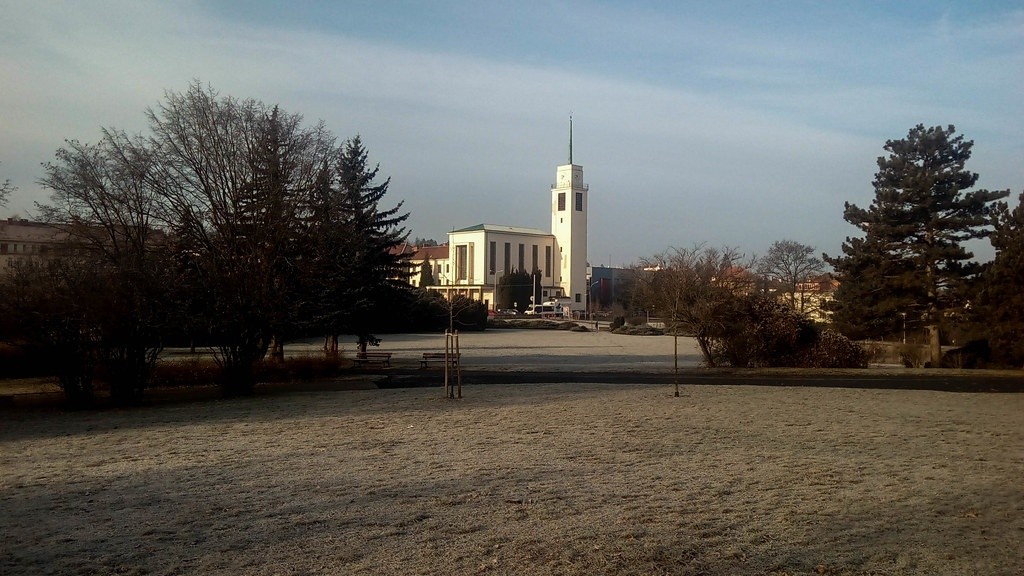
[902, 313, 907, 345]
[589, 281, 598, 320]
[490, 270, 504, 312]
[585, 294, 588, 322]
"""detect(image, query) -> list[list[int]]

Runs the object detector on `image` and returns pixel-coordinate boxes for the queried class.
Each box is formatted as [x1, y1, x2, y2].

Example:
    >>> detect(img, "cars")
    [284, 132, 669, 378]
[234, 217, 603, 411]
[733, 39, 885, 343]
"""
[498, 309, 519, 315]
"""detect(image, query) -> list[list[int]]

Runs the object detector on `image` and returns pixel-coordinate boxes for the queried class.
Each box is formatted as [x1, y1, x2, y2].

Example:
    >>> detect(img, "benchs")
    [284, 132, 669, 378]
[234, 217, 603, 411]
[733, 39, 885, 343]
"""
[417, 353, 461, 369]
[351, 352, 392, 368]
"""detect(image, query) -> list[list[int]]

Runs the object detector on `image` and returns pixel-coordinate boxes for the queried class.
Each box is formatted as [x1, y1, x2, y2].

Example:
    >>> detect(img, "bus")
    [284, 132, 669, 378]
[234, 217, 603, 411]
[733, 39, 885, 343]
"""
[524, 302, 555, 315]
